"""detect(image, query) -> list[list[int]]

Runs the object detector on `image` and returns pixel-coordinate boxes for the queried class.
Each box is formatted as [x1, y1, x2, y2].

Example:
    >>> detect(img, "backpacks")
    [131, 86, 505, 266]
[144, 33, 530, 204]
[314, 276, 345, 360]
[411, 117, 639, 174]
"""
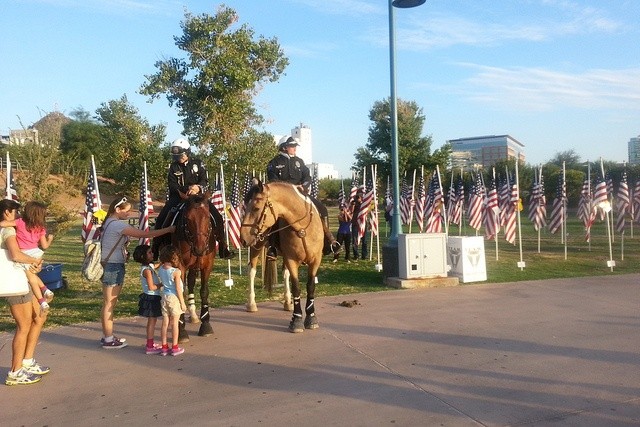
[81, 219, 123, 282]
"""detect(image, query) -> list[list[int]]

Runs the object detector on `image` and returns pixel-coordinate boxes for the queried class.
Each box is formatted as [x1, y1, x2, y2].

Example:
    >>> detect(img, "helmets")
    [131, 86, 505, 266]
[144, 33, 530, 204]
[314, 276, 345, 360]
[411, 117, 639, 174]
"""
[278, 135, 300, 153]
[171, 138, 191, 156]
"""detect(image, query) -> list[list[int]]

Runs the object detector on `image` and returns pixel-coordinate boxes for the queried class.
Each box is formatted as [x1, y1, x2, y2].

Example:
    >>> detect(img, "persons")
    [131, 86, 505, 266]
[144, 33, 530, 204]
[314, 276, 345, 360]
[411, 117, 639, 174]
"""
[1, 200, 56, 318]
[332, 203, 353, 263]
[387, 194, 392, 234]
[0, 199, 50, 385]
[100, 197, 177, 349]
[348, 192, 370, 261]
[265, 135, 342, 260]
[158, 245, 187, 356]
[150, 136, 237, 261]
[132, 243, 165, 355]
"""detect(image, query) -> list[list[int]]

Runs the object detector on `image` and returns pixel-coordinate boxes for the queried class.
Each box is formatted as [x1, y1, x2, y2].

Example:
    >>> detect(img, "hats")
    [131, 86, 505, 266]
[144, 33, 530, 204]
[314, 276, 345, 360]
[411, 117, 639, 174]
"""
[339, 203, 347, 208]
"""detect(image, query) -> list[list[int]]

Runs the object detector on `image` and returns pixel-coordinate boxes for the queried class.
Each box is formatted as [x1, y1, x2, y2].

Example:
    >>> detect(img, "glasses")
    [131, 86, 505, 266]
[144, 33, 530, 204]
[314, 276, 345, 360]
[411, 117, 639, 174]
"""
[114, 197, 127, 208]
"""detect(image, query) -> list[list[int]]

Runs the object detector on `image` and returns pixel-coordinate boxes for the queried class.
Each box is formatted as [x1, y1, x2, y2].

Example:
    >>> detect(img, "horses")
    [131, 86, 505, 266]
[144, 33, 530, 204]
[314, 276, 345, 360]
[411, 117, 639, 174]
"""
[153, 185, 217, 344]
[239, 178, 325, 333]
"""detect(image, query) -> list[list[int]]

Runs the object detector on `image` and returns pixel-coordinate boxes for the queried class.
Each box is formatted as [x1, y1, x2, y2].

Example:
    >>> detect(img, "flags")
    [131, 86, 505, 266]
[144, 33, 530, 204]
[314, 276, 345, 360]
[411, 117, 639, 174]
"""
[243, 170, 250, 200]
[549, 169, 568, 235]
[338, 180, 346, 207]
[137, 159, 154, 246]
[467, 172, 483, 230]
[604, 171, 614, 199]
[401, 172, 415, 224]
[415, 174, 426, 230]
[497, 175, 503, 205]
[468, 174, 476, 200]
[503, 167, 517, 245]
[309, 162, 319, 199]
[426, 170, 447, 233]
[630, 175, 640, 223]
[498, 175, 510, 225]
[81, 154, 103, 245]
[450, 176, 465, 224]
[586, 166, 612, 230]
[355, 167, 375, 246]
[369, 178, 379, 236]
[508, 175, 514, 193]
[209, 163, 225, 230]
[480, 175, 488, 200]
[616, 168, 629, 233]
[348, 166, 361, 206]
[529, 178, 537, 221]
[483, 175, 500, 239]
[400, 172, 409, 210]
[577, 174, 593, 242]
[447, 181, 456, 221]
[228, 167, 242, 250]
[534, 175, 547, 231]
[4, 150, 20, 205]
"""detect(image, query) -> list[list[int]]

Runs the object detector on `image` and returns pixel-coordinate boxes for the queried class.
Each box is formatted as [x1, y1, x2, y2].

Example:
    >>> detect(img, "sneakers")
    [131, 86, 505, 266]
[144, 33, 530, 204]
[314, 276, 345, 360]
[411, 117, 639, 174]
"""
[146, 347, 161, 355]
[161, 348, 173, 356]
[344, 259, 350, 263]
[171, 347, 185, 356]
[43, 289, 54, 304]
[361, 255, 366, 259]
[100, 334, 126, 345]
[333, 258, 338, 262]
[21, 359, 51, 376]
[39, 302, 49, 318]
[4, 369, 42, 386]
[353, 256, 359, 260]
[145, 343, 162, 349]
[102, 339, 127, 349]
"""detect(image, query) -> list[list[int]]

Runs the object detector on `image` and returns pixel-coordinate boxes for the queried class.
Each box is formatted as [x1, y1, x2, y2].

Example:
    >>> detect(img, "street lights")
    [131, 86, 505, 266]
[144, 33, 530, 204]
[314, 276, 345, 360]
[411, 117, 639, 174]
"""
[388, 0, 425, 245]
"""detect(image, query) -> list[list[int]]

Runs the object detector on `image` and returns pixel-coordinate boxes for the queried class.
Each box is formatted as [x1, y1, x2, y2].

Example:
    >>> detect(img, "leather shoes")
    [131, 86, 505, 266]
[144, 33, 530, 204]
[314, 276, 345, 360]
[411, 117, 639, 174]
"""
[323, 240, 342, 255]
[219, 250, 235, 258]
[267, 245, 279, 257]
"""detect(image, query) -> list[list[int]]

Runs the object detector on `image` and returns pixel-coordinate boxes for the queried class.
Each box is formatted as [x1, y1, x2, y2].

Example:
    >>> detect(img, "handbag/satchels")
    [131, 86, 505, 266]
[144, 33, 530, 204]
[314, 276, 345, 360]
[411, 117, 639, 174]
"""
[0, 225, 29, 297]
